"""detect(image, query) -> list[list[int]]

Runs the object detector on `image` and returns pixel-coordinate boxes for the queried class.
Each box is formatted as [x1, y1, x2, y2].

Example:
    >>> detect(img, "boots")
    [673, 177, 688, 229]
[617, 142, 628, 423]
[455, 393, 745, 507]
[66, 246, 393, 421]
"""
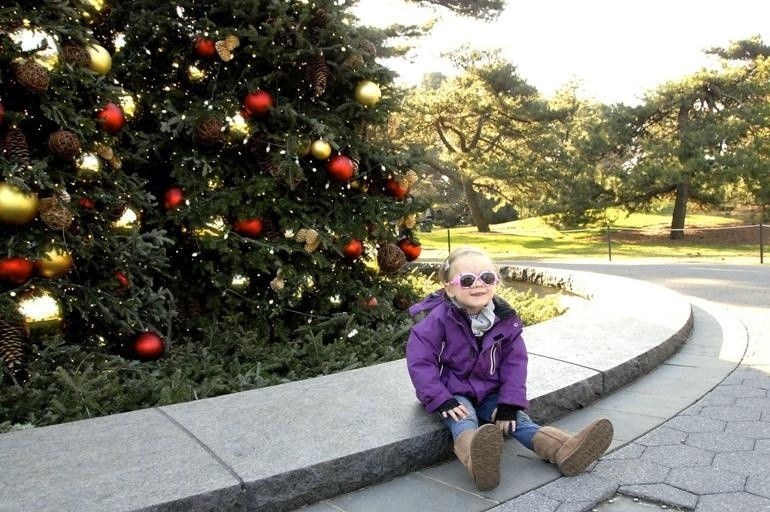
[453, 423, 504, 492]
[530, 417, 613, 477]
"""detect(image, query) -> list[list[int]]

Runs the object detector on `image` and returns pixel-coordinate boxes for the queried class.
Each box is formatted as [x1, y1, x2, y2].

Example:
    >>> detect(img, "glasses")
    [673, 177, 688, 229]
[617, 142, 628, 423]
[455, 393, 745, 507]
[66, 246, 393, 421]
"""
[444, 269, 498, 290]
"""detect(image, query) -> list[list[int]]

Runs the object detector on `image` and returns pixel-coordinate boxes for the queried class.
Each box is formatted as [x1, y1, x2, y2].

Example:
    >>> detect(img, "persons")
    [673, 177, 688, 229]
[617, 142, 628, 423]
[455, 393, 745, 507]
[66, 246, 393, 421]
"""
[404, 244, 614, 491]
